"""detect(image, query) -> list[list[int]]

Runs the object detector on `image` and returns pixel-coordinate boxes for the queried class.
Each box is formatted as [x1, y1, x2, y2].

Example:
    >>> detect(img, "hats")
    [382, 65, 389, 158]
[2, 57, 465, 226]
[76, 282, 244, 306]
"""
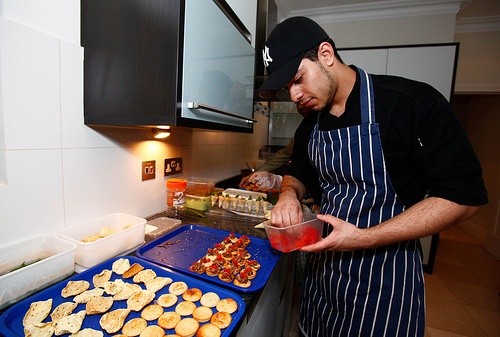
[256, 16, 330, 93]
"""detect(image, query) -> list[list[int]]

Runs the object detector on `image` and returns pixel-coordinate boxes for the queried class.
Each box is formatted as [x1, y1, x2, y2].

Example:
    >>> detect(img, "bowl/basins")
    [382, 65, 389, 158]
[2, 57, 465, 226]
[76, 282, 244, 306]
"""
[264, 212, 324, 253]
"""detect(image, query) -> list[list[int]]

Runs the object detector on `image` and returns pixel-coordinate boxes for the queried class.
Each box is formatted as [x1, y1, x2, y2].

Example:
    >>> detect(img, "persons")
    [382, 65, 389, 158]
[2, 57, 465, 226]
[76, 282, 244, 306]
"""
[238, 100, 322, 286]
[259, 15, 490, 337]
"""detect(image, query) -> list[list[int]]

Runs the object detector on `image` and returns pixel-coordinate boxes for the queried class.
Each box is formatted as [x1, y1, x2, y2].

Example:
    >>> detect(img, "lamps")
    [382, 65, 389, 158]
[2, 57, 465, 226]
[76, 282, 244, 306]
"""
[153, 128, 171, 138]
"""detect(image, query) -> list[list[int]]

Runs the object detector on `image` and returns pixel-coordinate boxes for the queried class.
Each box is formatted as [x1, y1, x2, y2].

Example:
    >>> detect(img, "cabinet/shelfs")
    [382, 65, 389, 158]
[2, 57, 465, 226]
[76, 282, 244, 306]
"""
[80, 0, 260, 133]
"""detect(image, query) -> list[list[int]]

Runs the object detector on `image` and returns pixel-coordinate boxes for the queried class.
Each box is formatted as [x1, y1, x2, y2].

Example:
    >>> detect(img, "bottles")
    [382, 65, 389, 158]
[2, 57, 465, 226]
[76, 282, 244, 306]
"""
[167, 181, 187, 220]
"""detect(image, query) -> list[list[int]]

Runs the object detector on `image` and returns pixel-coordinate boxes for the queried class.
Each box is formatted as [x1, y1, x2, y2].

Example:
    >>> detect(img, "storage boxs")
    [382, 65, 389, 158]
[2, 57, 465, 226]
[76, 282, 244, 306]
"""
[0, 213, 147, 310]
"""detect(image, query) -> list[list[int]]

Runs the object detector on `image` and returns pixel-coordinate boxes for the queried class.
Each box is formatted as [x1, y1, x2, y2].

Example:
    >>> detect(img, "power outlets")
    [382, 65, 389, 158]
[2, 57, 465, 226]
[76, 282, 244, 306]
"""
[164, 157, 182, 176]
